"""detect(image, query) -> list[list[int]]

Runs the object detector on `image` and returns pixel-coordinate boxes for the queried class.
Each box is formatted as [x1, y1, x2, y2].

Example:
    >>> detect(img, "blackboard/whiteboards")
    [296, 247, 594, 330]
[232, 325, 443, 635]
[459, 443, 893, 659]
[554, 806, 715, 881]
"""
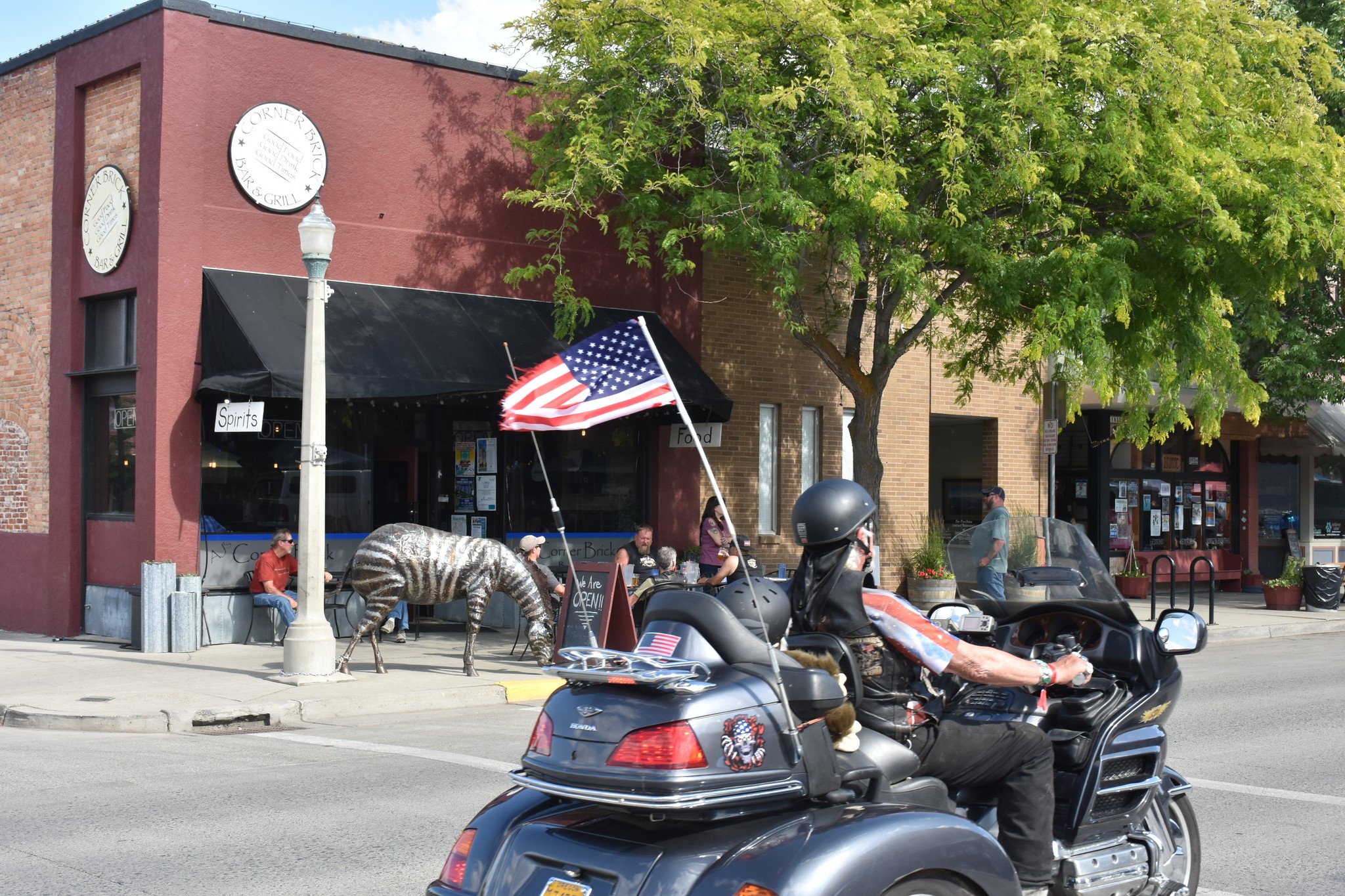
[553, 562, 638, 665]
[1286, 528, 1304, 575]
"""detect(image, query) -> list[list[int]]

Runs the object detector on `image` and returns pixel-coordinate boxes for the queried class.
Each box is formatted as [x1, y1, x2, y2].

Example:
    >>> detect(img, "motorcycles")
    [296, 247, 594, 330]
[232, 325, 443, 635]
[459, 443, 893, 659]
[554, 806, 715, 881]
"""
[422, 515, 1207, 896]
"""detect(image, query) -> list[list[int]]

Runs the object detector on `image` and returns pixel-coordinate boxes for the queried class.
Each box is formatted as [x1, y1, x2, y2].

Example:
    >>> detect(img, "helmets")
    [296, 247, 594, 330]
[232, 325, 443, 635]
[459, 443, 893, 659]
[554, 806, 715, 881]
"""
[791, 478, 877, 547]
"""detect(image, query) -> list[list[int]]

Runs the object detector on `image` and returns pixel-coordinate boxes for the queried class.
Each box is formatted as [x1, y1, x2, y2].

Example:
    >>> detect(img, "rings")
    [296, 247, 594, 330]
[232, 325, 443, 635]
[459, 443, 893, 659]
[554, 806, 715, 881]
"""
[1085, 662, 1094, 675]
[1070, 651, 1080, 658]
[329, 576, 331, 577]
[1080, 655, 1088, 662]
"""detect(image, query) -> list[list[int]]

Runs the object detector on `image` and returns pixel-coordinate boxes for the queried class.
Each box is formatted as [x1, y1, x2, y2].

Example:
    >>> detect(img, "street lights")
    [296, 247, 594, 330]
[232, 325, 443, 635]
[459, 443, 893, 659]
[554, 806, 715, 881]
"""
[281, 191, 340, 677]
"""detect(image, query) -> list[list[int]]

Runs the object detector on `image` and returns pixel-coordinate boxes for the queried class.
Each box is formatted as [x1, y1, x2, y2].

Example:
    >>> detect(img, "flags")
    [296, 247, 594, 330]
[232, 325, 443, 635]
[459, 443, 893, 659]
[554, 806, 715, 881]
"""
[498, 318, 678, 430]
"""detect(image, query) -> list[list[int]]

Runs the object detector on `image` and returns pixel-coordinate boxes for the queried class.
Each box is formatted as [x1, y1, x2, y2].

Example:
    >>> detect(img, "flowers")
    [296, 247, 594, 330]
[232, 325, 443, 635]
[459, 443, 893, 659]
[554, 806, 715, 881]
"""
[1111, 555, 1145, 578]
[919, 566, 956, 580]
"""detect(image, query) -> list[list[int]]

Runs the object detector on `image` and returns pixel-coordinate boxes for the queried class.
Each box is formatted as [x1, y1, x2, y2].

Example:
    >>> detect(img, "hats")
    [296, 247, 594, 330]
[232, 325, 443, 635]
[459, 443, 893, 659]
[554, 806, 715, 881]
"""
[731, 535, 755, 551]
[519, 535, 546, 553]
[981, 486, 1005, 500]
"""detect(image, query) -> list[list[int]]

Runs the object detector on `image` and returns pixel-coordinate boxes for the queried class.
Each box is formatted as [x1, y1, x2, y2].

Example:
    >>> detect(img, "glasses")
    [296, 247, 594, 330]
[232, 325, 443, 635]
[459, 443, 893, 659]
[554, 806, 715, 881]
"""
[535, 544, 541, 548]
[730, 545, 732, 548]
[986, 493, 996, 497]
[279, 539, 293, 544]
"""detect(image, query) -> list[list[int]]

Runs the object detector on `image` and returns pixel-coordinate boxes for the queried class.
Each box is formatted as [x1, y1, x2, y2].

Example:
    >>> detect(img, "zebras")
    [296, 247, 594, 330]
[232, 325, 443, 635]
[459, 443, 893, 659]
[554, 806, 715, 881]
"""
[334, 521, 558, 680]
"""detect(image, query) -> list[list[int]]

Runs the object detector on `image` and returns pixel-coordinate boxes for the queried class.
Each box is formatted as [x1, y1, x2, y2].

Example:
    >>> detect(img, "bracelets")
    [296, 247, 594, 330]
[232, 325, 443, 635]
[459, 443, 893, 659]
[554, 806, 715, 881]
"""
[705, 577, 710, 582]
[1030, 657, 1057, 712]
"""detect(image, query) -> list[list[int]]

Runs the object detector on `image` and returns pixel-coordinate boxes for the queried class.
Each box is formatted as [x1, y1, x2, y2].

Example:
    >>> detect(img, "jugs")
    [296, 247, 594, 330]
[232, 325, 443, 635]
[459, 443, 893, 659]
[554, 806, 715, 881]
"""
[686, 561, 700, 584]
[623, 564, 635, 586]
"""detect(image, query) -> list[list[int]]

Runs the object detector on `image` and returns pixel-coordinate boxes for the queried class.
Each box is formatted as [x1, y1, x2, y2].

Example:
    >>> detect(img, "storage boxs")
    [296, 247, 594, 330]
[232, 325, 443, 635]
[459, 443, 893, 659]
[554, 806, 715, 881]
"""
[516, 664, 802, 797]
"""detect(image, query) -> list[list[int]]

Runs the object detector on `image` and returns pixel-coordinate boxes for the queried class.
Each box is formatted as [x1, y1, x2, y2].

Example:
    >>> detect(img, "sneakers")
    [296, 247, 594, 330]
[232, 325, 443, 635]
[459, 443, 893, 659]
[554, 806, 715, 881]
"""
[380, 619, 395, 634]
[396, 631, 406, 643]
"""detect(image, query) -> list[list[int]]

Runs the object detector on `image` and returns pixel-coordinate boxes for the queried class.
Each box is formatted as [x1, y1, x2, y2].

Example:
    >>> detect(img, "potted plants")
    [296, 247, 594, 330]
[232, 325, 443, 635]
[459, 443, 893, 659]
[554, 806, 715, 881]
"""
[1260, 556, 1306, 611]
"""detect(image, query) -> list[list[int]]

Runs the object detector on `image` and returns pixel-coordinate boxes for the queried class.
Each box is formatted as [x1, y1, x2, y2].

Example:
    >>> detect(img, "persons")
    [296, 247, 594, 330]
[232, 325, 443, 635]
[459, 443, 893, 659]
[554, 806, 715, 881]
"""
[614, 524, 660, 608]
[380, 600, 409, 643]
[518, 534, 566, 625]
[628, 545, 685, 629]
[699, 496, 732, 598]
[250, 528, 333, 647]
[969, 486, 1010, 604]
[790, 480, 1094, 896]
[697, 534, 764, 587]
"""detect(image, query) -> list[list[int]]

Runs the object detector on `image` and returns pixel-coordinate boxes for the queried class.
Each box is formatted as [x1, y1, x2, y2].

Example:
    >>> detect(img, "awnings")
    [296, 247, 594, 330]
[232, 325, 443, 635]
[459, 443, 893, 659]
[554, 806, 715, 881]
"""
[194, 265, 737, 428]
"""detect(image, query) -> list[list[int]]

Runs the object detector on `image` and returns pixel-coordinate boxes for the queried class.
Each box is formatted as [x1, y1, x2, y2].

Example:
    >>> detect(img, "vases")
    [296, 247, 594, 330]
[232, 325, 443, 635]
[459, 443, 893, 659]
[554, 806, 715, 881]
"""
[906, 576, 957, 617]
[1002, 575, 1046, 603]
[1240, 574, 1263, 588]
[1114, 576, 1150, 599]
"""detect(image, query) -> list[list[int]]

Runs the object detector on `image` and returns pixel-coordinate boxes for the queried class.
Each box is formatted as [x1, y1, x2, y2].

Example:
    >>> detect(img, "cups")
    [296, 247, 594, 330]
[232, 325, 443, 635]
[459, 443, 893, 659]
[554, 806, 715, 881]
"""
[718, 568, 727, 583]
[717, 547, 729, 561]
[779, 563, 786, 579]
[650, 569, 659, 576]
[680, 562, 687, 583]
[631, 574, 640, 586]
[761, 564, 766, 577]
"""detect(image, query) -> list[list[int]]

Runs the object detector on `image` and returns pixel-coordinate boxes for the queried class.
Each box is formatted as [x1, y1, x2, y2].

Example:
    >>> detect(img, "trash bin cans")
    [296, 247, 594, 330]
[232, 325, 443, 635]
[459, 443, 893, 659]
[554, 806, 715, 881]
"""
[1302, 565, 1341, 612]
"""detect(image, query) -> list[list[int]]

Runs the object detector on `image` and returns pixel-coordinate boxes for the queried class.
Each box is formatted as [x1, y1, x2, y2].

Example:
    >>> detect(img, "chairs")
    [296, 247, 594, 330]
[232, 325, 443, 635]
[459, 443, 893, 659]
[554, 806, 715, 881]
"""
[703, 584, 729, 598]
[243, 570, 297, 648]
[633, 581, 688, 640]
[508, 588, 563, 661]
[765, 568, 798, 578]
[322, 557, 422, 641]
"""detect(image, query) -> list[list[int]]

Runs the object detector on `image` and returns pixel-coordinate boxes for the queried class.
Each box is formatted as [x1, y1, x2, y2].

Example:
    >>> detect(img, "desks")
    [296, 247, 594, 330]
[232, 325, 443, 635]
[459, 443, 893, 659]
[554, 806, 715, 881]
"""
[626, 581, 728, 598]
[324, 572, 353, 639]
[763, 577, 792, 584]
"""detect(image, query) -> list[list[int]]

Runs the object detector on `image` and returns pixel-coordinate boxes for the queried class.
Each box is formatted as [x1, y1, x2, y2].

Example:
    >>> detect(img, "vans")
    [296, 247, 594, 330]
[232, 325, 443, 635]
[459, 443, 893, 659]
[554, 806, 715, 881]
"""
[271, 469, 370, 534]
